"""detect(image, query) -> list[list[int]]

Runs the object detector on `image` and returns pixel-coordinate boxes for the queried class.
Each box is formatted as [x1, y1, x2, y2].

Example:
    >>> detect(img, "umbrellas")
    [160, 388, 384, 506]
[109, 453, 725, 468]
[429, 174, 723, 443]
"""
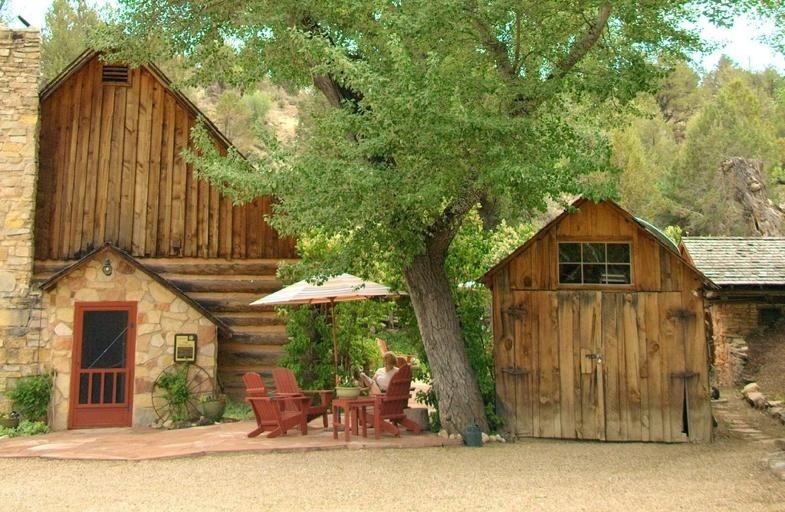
[247, 268, 409, 385]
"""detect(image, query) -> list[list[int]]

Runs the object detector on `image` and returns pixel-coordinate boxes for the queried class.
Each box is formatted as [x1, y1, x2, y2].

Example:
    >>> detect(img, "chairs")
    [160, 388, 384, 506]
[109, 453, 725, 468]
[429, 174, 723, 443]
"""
[241, 372, 314, 439]
[273, 367, 334, 428]
[357, 365, 423, 437]
[396, 357, 406, 368]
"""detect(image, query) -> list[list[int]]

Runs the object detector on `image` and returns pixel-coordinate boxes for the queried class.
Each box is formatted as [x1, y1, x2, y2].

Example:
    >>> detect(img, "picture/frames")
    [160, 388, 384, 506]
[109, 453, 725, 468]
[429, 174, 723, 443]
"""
[173, 334, 197, 365]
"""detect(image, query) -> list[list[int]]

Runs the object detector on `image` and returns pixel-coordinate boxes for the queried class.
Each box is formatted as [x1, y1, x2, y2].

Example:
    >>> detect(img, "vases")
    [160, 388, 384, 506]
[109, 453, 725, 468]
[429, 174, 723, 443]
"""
[0, 419, 18, 428]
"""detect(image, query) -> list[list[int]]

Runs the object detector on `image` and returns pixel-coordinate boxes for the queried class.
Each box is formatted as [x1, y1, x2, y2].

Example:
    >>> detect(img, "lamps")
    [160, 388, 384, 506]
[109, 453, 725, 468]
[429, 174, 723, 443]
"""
[102, 258, 112, 276]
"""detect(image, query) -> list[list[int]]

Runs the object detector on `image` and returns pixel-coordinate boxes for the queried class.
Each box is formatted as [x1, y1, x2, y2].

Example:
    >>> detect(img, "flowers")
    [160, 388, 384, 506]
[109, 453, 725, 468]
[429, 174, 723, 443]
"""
[0, 411, 19, 418]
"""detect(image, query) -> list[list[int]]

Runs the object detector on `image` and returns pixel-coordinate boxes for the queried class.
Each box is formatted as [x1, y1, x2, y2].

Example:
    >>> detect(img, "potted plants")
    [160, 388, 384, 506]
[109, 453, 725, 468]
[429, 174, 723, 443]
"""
[198, 394, 226, 419]
[335, 381, 361, 399]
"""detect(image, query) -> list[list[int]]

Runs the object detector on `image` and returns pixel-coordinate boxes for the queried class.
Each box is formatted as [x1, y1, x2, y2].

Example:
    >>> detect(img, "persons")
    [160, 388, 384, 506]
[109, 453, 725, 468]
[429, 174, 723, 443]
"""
[353, 365, 376, 396]
[372, 351, 400, 393]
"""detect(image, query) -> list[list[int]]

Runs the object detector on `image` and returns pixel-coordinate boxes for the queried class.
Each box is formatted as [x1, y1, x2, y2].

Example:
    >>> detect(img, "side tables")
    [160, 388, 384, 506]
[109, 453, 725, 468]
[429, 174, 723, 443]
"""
[332, 396, 382, 442]
